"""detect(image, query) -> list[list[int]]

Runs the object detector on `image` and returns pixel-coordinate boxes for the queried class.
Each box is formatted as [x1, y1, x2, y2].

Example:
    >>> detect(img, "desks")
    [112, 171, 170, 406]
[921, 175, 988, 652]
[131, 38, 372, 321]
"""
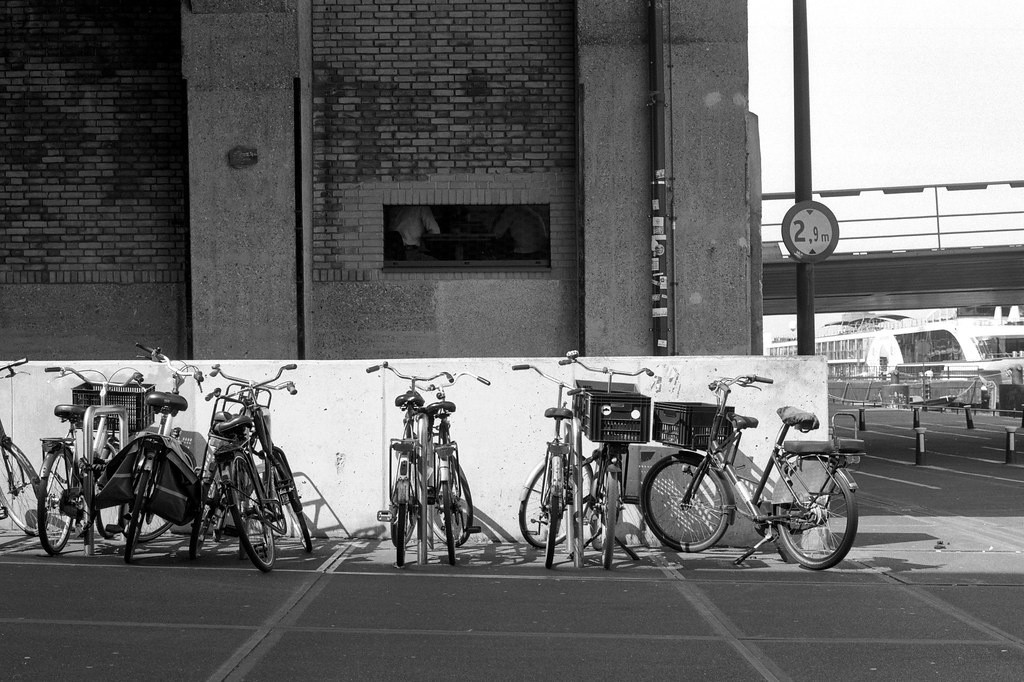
[422, 233, 497, 261]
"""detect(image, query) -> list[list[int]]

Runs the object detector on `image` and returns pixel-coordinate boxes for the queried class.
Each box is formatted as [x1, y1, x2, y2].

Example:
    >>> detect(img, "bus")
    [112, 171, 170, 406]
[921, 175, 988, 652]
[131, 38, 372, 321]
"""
[895, 358, 1024, 406]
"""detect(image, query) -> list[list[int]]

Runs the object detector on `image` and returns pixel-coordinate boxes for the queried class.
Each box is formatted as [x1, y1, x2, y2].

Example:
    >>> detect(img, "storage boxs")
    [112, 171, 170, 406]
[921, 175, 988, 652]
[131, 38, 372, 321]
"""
[71, 382, 155, 433]
[573, 379, 650, 444]
[654, 402, 734, 448]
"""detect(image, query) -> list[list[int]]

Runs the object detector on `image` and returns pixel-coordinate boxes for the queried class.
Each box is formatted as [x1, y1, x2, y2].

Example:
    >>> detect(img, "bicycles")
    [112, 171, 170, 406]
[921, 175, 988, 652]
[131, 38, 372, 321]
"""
[510, 348, 658, 575]
[0, 339, 314, 573]
[364, 361, 492, 569]
[640, 373, 859, 571]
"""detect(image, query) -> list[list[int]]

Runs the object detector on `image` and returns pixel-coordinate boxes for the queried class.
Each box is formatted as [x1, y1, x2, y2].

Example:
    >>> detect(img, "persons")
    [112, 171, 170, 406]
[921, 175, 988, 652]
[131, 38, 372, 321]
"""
[389, 204, 442, 259]
[493, 204, 547, 261]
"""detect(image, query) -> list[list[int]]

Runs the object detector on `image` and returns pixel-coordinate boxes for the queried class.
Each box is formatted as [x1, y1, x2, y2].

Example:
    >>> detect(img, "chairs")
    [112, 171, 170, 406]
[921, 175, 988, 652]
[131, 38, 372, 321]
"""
[383, 231, 408, 261]
